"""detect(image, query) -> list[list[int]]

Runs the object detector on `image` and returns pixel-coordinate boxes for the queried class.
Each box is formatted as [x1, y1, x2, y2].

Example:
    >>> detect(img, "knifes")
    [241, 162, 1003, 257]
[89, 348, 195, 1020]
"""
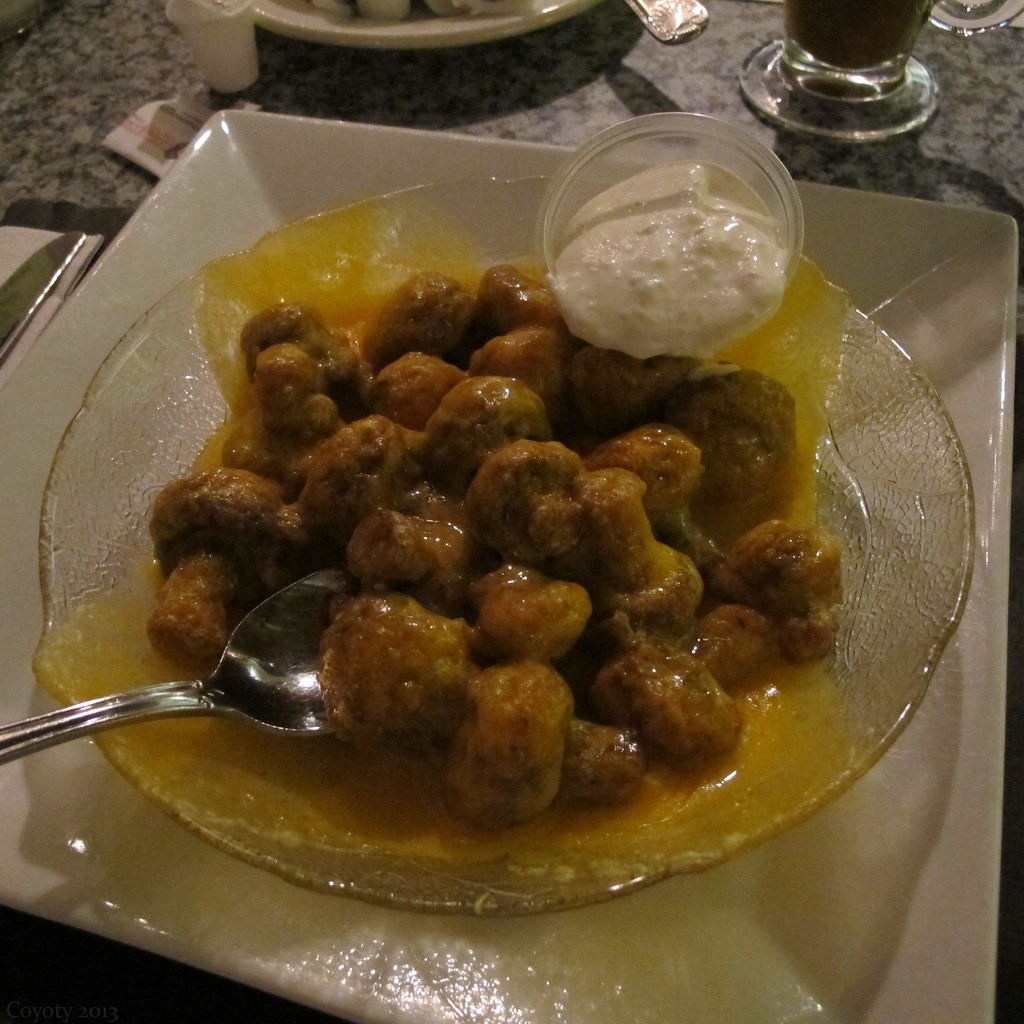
[0, 230, 86, 359]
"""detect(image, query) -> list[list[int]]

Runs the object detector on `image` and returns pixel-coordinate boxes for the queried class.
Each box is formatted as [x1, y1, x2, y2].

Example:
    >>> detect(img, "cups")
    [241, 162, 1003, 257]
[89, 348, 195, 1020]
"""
[740, 0, 940, 140]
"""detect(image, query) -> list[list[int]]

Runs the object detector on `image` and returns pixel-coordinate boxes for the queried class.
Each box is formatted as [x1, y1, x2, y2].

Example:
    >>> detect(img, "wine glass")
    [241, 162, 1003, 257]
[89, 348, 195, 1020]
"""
[929, 0, 1024, 35]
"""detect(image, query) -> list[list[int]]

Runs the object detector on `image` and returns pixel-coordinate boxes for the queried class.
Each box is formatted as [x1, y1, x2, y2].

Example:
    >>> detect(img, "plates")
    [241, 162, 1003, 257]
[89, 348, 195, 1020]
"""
[32, 168, 978, 915]
[33, 176, 977, 915]
[0, 110, 1017, 1024]
[252, 0, 602, 48]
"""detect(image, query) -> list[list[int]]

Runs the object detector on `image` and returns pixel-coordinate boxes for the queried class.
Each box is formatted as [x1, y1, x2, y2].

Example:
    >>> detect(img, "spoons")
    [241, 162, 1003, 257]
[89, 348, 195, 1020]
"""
[0, 563, 354, 758]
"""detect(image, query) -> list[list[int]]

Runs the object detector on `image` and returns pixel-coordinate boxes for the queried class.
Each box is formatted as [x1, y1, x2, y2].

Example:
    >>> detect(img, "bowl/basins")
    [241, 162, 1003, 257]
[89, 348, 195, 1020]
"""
[538, 112, 804, 292]
